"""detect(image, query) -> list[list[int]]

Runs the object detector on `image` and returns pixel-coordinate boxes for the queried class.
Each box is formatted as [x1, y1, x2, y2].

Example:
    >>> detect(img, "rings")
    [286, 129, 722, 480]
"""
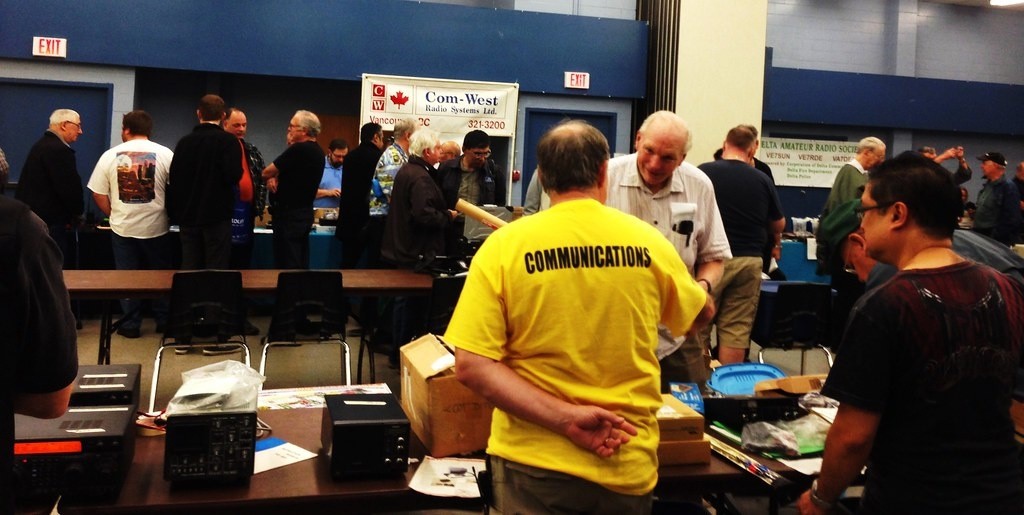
[606, 439, 608, 442]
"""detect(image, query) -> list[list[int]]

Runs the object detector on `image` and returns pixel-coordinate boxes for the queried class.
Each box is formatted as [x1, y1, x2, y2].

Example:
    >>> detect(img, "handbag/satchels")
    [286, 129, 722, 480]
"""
[232, 183, 251, 243]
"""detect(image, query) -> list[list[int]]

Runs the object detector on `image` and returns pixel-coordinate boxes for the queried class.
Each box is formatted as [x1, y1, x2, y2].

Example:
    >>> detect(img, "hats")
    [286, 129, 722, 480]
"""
[976, 151, 1006, 166]
[815, 199, 867, 274]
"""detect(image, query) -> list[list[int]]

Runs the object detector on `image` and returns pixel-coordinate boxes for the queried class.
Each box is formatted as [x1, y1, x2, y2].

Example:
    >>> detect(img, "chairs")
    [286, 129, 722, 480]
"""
[148, 270, 251, 412]
[260, 271, 351, 389]
[749, 282, 838, 370]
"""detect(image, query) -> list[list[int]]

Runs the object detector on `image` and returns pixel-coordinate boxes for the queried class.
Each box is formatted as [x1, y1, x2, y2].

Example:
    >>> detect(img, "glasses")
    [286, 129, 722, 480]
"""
[852, 203, 894, 221]
[843, 244, 855, 273]
[66, 121, 81, 128]
[471, 149, 491, 158]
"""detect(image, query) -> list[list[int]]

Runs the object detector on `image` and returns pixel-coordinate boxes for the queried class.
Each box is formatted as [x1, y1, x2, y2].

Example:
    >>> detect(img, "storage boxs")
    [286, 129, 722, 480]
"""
[401, 333, 495, 458]
[655, 393, 705, 442]
[656, 439, 711, 467]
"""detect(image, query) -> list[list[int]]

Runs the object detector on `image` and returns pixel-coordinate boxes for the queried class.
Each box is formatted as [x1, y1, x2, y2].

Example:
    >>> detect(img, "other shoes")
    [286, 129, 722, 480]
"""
[175, 342, 199, 354]
[117, 328, 140, 338]
[202, 340, 241, 354]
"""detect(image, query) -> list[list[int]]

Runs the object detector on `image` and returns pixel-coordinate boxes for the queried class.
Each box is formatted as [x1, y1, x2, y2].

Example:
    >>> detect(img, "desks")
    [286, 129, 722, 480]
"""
[167, 222, 348, 316]
[62, 270, 434, 385]
[775, 238, 833, 283]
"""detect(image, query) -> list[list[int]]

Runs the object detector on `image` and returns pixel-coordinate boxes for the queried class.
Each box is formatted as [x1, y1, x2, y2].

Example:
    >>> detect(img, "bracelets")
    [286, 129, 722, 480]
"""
[962, 156, 966, 162]
[810, 479, 831, 510]
[774, 244, 782, 248]
[697, 279, 711, 292]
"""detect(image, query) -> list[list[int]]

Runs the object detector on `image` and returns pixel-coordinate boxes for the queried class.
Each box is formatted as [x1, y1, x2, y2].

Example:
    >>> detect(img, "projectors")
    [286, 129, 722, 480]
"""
[10, 364, 142, 511]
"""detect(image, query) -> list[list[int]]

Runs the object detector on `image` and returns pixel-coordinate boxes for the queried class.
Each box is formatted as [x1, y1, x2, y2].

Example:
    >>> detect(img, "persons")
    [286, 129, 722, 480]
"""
[442, 119, 717, 515]
[14, 93, 1024, 368]
[609, 110, 733, 382]
[0, 147, 80, 515]
[796, 151, 1024, 515]
